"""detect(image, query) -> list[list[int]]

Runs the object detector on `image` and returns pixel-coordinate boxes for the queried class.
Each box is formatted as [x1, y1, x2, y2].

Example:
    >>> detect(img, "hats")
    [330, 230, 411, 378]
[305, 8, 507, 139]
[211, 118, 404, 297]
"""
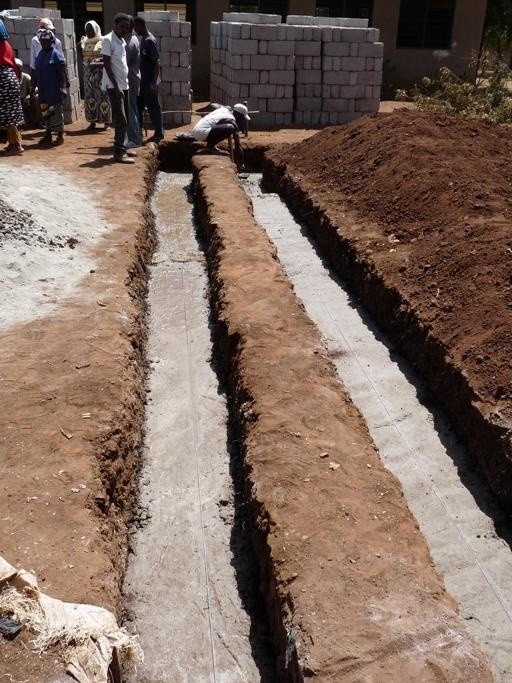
[39, 19, 56, 31]
[233, 103, 251, 121]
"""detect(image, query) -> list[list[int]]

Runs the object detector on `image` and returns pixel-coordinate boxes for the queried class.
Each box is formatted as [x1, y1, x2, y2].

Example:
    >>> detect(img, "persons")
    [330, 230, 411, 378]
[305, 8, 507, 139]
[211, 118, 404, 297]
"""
[191, 102, 252, 169]
[0, 8, 164, 165]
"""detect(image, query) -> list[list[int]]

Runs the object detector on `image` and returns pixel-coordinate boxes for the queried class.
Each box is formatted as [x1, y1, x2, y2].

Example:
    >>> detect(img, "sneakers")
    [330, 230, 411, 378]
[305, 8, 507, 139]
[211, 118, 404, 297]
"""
[3, 137, 64, 156]
[113, 141, 141, 164]
